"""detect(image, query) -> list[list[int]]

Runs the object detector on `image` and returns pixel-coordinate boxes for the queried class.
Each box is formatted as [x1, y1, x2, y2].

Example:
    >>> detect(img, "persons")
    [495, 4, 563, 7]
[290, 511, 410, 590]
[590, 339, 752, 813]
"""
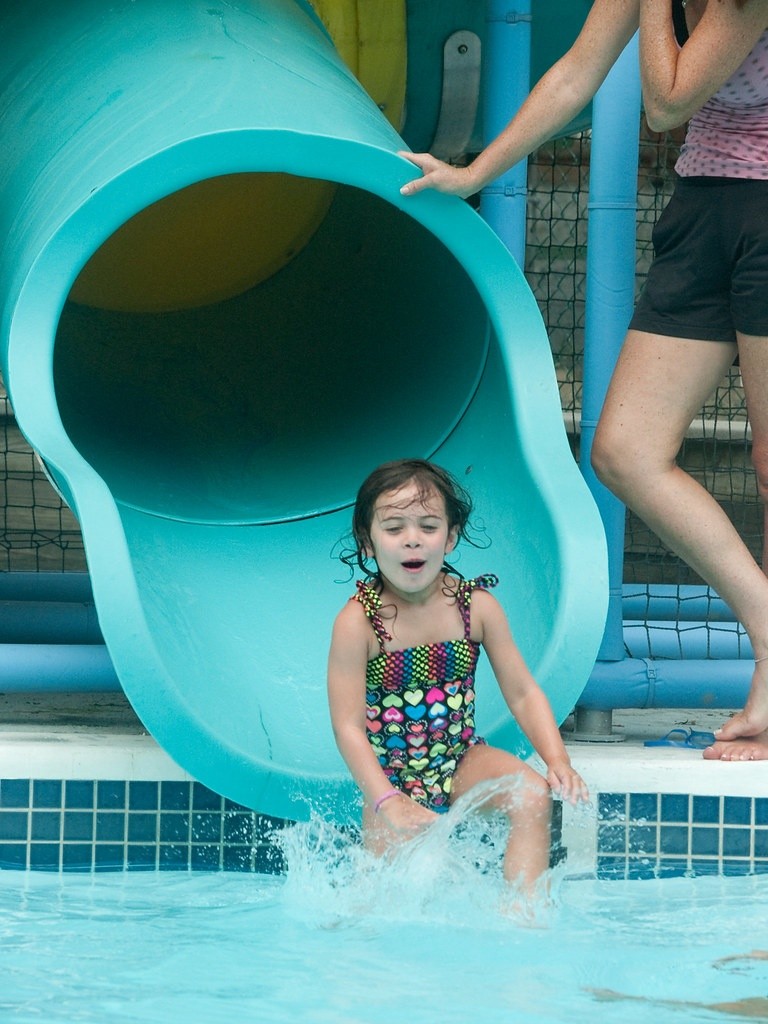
[396, 0, 768, 761]
[327, 458, 589, 890]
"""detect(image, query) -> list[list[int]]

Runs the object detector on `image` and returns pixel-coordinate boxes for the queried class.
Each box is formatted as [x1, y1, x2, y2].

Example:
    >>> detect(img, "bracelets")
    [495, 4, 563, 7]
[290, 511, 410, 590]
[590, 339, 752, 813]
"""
[374, 791, 400, 813]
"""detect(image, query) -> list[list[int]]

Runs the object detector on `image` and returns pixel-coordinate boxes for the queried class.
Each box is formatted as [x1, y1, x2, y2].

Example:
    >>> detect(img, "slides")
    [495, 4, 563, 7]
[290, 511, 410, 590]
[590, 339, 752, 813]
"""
[0, 0, 612, 830]
